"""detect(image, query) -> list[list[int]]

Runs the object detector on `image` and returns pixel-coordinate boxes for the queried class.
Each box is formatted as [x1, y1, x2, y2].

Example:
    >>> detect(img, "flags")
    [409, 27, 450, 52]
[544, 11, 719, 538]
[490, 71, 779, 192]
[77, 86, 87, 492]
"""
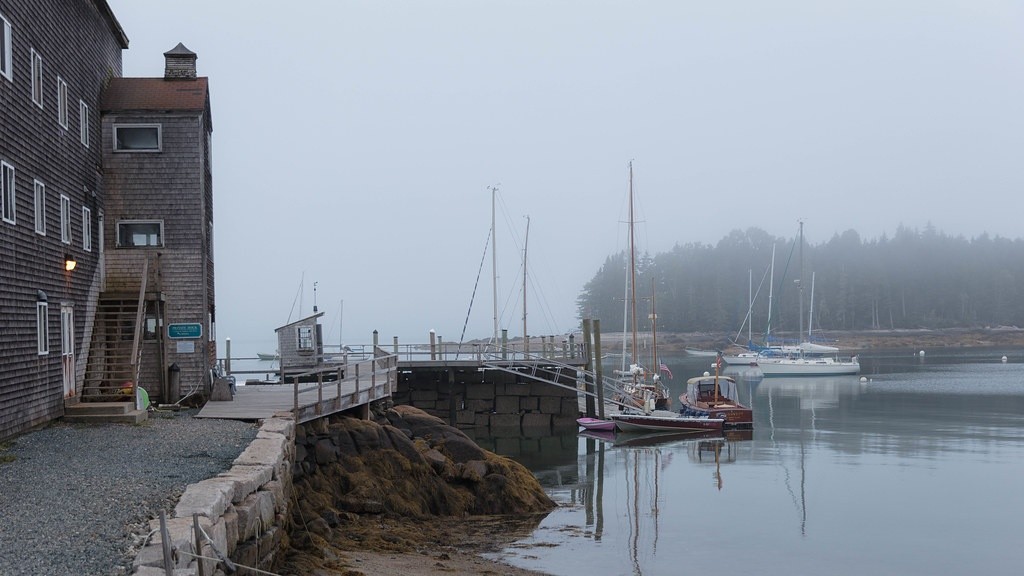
[660, 363, 674, 380]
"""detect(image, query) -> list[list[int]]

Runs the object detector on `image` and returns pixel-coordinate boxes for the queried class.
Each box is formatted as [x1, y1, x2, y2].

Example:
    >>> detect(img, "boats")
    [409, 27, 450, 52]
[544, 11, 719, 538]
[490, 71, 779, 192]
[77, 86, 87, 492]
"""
[576, 417, 617, 432]
[609, 400, 726, 433]
[677, 348, 755, 430]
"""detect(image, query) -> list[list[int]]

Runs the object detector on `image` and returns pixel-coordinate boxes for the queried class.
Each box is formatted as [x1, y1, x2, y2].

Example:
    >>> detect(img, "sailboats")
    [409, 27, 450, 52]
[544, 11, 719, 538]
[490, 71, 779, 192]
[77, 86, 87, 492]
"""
[683, 217, 862, 378]
[452, 185, 597, 396]
[323, 299, 372, 362]
[608, 159, 673, 412]
[255, 272, 306, 361]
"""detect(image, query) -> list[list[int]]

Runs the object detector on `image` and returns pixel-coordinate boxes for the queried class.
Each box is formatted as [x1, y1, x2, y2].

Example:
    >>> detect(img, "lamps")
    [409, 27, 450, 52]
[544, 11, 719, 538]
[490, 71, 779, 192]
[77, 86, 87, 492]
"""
[65, 254, 76, 271]
